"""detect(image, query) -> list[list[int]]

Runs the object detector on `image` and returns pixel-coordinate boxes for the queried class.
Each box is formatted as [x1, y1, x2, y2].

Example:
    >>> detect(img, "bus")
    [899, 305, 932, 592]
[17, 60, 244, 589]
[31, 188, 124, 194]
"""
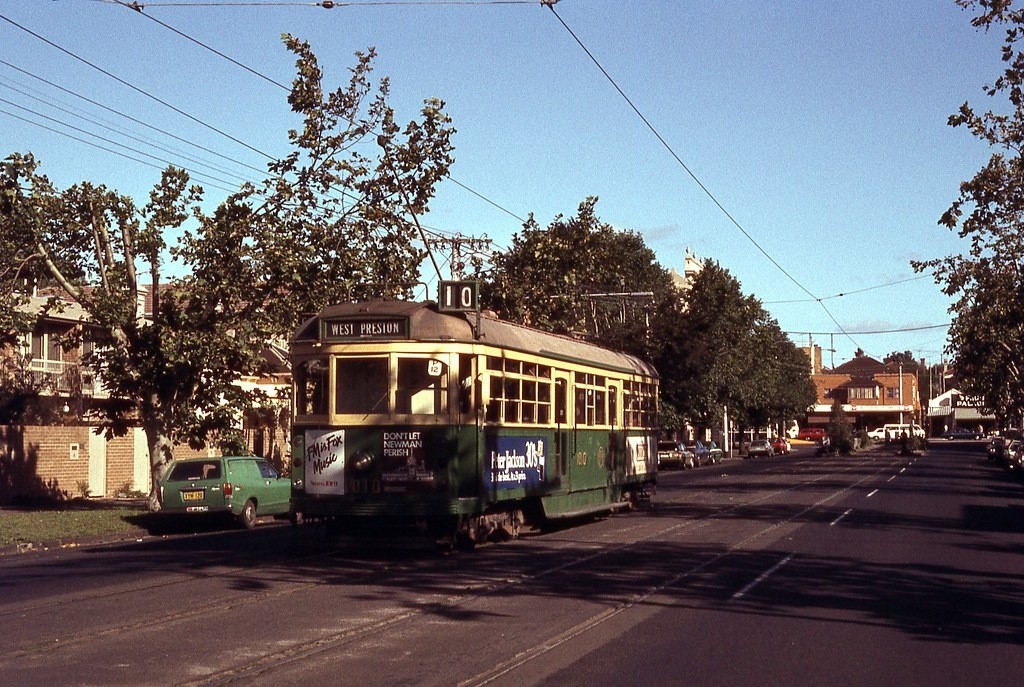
[285, 277, 663, 553]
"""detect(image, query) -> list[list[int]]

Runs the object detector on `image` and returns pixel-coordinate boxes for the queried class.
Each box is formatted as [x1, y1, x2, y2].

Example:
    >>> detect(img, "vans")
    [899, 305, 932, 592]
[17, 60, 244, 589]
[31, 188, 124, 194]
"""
[882, 423, 926, 440]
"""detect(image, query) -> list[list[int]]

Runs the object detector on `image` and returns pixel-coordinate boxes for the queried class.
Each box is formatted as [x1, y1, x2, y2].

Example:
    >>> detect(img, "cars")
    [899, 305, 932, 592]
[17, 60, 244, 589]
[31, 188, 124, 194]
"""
[657, 437, 724, 469]
[797, 427, 832, 441]
[941, 425, 985, 440]
[746, 437, 792, 459]
[866, 427, 883, 441]
[984, 435, 1024, 475]
[148, 455, 291, 530]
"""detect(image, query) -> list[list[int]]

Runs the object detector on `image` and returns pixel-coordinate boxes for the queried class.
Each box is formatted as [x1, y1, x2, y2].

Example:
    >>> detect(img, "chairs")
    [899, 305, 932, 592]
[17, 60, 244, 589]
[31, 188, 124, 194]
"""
[207, 468, 215, 478]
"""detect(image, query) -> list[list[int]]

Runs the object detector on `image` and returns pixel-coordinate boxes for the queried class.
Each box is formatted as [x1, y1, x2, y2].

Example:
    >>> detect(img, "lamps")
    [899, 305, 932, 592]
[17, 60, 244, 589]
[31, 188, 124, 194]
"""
[62, 401, 70, 413]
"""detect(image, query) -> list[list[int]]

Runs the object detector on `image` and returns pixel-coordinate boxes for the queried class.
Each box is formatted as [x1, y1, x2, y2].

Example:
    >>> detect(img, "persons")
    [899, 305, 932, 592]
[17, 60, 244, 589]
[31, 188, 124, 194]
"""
[885, 430, 891, 446]
[901, 429, 906, 452]
[978, 424, 983, 433]
